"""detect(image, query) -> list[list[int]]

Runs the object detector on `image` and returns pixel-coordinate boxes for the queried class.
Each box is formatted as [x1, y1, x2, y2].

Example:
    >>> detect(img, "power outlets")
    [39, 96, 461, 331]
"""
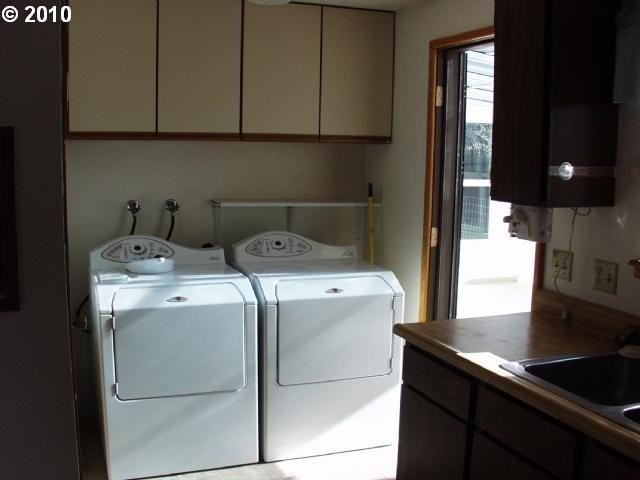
[592, 258, 619, 296]
[551, 247, 574, 281]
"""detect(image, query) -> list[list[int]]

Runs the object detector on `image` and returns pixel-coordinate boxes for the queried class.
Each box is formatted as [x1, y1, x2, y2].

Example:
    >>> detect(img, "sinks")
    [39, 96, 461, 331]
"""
[588, 407, 639, 434]
[498, 347, 640, 407]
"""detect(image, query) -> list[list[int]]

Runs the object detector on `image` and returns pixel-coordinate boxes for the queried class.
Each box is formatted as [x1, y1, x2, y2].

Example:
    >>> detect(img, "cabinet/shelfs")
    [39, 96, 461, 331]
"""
[322, 6, 396, 135]
[158, 2, 242, 135]
[490, 1, 619, 207]
[392, 343, 577, 479]
[242, 0, 322, 134]
[577, 433, 639, 479]
[69, 0, 157, 133]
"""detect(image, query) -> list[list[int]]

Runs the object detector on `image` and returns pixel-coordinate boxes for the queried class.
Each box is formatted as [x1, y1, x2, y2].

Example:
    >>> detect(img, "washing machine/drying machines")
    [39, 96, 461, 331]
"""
[88, 235, 261, 480]
[231, 231, 405, 462]
